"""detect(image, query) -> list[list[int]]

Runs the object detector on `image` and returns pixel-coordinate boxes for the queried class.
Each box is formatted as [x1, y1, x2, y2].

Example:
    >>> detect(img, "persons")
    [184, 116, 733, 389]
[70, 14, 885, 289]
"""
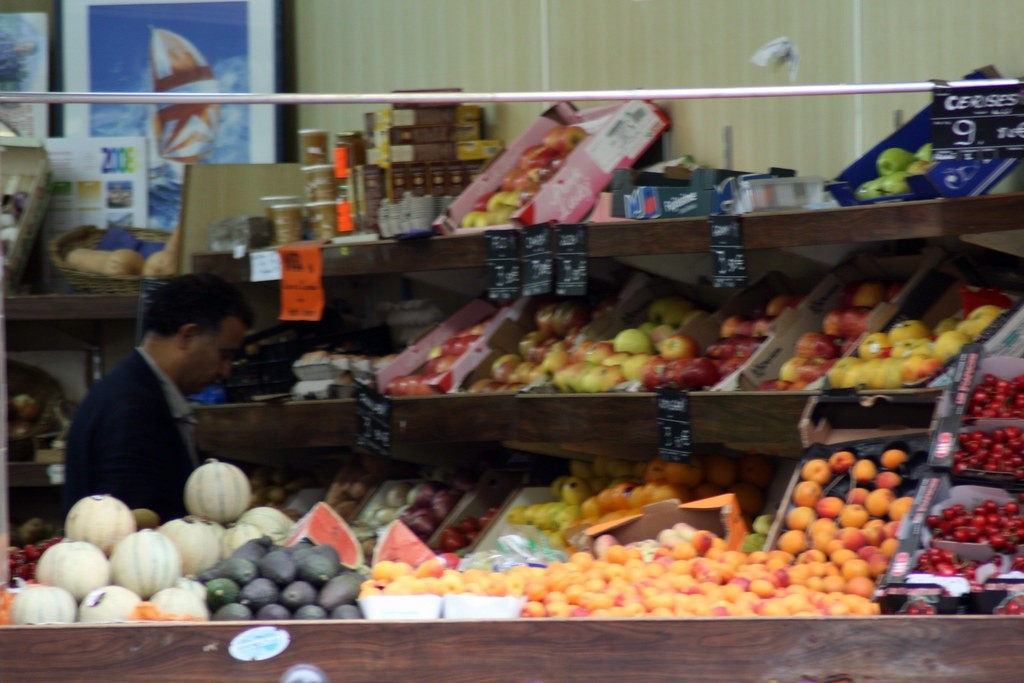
[66, 272, 277, 536]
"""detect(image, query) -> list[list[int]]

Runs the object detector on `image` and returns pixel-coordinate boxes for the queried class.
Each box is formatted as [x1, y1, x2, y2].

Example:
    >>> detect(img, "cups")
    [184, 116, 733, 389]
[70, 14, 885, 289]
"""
[269, 204, 300, 243]
[259, 195, 302, 221]
[298, 127, 330, 167]
[302, 163, 341, 207]
[306, 201, 337, 241]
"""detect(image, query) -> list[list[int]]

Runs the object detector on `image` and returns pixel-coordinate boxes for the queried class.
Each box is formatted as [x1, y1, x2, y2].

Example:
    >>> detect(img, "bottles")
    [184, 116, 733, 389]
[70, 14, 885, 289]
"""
[336, 184, 357, 235]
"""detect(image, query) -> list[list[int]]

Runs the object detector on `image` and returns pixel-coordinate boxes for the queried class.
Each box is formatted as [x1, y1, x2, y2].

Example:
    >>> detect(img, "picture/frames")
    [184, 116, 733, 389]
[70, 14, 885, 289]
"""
[0, 0, 65, 138]
[54, 0, 286, 236]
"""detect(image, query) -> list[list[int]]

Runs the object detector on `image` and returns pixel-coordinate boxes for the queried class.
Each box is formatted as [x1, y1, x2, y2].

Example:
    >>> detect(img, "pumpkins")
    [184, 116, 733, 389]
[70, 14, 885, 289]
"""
[10, 458, 294, 625]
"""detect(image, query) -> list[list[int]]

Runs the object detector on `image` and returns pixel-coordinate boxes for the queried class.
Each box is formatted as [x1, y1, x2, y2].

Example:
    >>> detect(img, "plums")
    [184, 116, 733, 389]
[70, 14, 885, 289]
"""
[358, 449, 914, 620]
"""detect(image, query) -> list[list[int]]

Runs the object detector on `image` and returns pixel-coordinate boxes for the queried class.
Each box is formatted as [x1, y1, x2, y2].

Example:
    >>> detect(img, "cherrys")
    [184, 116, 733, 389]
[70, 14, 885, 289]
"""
[907, 373, 1024, 616]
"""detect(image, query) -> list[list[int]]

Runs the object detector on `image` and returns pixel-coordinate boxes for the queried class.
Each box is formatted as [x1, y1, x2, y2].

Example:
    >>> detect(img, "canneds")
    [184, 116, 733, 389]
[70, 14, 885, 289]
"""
[337, 133, 365, 179]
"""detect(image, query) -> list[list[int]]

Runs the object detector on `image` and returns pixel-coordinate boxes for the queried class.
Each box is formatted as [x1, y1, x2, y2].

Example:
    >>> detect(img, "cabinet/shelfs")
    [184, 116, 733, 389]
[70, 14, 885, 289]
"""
[163, 154, 1024, 592]
[4, 293, 158, 488]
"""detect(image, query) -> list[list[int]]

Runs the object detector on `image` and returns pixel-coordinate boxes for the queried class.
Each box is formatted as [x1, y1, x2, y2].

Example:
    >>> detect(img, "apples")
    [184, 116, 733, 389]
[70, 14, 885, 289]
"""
[458, 125, 588, 229]
[380, 279, 1002, 394]
[853, 141, 930, 201]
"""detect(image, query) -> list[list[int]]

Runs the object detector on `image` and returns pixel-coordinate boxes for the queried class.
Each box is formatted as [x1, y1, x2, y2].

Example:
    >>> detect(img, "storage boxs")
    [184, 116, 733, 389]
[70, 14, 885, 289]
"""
[612, 167, 798, 220]
[365, 88, 504, 234]
[183, 240, 1023, 625]
[0, 136, 56, 293]
[820, 64, 1023, 208]
[747, 174, 827, 213]
[448, 99, 672, 229]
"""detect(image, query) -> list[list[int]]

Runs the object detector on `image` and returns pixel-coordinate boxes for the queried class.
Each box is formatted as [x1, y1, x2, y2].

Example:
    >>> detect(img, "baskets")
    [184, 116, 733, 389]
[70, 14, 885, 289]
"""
[7, 359, 65, 461]
[49, 226, 173, 294]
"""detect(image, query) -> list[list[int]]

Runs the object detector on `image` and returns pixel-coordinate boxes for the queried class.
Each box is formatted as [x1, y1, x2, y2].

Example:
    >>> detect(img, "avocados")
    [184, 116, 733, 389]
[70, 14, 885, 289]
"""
[195, 539, 362, 620]
